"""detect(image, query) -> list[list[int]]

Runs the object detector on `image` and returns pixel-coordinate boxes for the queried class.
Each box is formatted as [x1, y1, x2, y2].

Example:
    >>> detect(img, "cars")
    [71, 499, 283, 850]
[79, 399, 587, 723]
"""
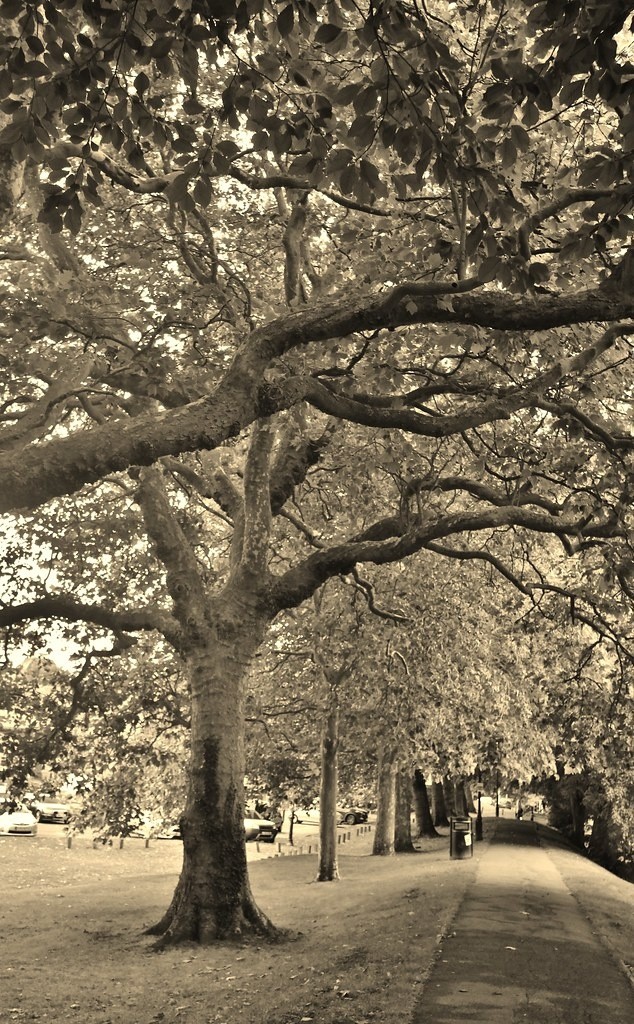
[334, 802, 369, 825]
[244, 807, 280, 843]
[288, 795, 343, 827]
[0, 797, 38, 837]
[127, 809, 179, 841]
[23, 790, 73, 825]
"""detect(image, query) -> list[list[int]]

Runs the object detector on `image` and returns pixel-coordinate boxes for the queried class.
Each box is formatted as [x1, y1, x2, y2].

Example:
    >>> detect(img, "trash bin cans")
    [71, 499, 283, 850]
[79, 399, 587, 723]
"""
[449, 816, 473, 860]
